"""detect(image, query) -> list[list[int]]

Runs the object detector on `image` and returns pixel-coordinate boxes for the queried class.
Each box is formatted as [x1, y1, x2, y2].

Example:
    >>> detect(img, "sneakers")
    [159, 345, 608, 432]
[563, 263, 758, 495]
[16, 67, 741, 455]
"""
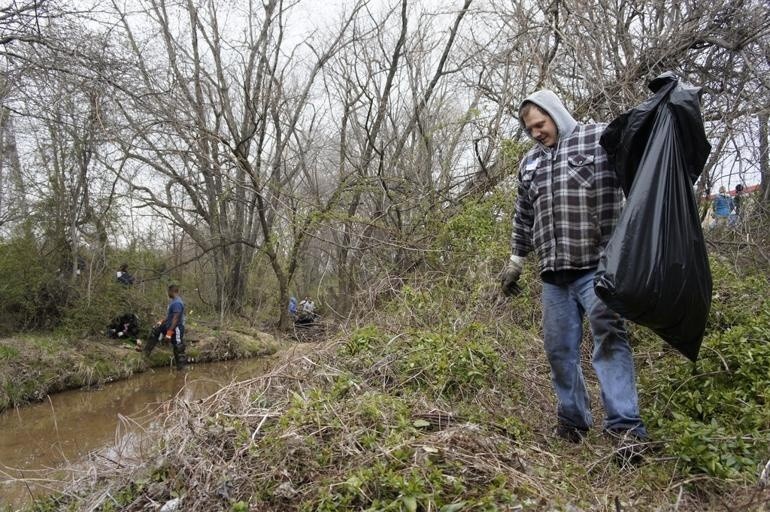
[612, 433, 640, 464]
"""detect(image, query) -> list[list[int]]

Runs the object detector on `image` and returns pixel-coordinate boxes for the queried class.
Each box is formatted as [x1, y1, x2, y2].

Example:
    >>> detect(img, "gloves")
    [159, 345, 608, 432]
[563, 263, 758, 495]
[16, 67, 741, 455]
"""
[500, 259, 522, 297]
[164, 328, 174, 344]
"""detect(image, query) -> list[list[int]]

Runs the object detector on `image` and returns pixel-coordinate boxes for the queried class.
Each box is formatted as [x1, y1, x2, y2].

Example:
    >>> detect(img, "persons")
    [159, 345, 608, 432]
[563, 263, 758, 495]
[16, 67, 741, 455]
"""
[713, 185, 734, 226]
[289, 296, 315, 314]
[117, 263, 135, 285]
[142, 280, 186, 370]
[733, 184, 749, 217]
[107, 312, 140, 339]
[497, 90, 648, 462]
